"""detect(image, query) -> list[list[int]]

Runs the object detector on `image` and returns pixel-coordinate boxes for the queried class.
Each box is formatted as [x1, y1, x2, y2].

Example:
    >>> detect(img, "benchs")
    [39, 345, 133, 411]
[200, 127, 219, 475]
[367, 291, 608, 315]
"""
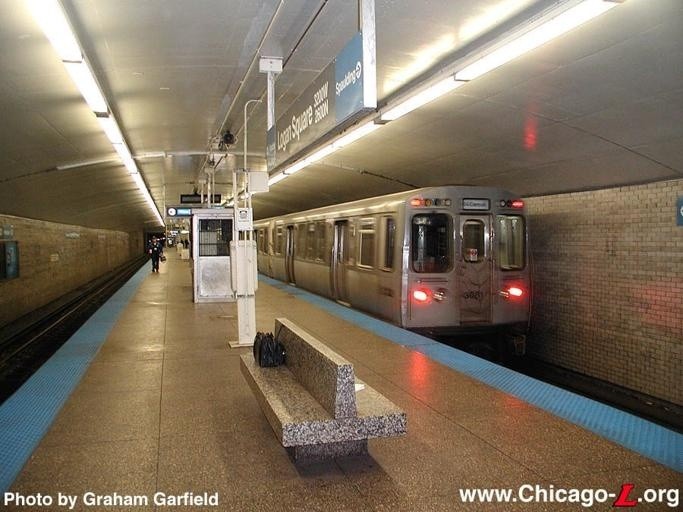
[238, 318, 407, 460]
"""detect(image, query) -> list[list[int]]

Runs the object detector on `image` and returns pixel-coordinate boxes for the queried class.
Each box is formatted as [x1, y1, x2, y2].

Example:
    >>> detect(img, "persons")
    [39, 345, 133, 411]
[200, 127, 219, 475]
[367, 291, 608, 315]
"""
[147, 235, 162, 273]
[181, 237, 189, 248]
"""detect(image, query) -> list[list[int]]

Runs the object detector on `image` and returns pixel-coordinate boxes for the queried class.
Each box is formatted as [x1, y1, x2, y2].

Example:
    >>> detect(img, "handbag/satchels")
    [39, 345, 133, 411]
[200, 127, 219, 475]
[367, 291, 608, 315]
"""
[254, 331, 288, 368]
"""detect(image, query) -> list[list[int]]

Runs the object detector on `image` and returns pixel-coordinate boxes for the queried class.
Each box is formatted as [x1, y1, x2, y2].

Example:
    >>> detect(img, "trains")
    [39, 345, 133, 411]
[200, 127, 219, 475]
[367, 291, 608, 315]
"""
[239, 186, 533, 329]
